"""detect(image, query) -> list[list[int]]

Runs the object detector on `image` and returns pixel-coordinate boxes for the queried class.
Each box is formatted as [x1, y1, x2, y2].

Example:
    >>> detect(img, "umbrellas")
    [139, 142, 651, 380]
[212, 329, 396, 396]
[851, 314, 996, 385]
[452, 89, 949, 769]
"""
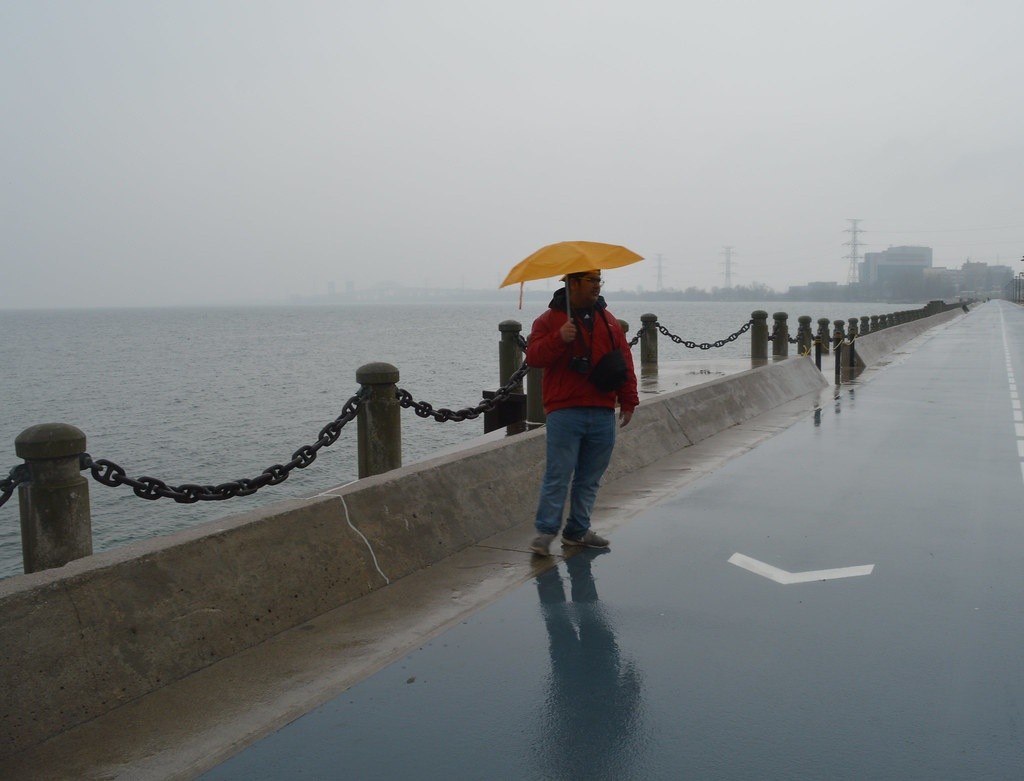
[500, 241, 645, 320]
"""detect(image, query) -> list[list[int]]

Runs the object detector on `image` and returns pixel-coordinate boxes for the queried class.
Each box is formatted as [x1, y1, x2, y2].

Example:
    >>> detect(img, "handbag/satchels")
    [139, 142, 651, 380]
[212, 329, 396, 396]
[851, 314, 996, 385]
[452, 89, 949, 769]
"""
[570, 302, 628, 392]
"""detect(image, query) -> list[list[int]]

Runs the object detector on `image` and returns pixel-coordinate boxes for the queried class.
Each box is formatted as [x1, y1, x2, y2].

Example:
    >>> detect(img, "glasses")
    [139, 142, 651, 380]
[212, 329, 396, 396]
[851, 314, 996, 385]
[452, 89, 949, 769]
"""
[573, 275, 606, 289]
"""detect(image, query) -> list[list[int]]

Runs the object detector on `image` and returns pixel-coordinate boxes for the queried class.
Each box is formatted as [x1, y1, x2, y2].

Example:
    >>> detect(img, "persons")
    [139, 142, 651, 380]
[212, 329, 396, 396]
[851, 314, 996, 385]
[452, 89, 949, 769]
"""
[525, 268, 640, 557]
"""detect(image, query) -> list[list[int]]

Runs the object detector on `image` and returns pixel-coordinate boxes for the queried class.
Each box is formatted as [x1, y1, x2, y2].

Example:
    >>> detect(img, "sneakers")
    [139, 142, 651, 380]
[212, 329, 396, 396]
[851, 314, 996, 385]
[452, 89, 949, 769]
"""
[528, 531, 555, 555]
[560, 528, 610, 549]
